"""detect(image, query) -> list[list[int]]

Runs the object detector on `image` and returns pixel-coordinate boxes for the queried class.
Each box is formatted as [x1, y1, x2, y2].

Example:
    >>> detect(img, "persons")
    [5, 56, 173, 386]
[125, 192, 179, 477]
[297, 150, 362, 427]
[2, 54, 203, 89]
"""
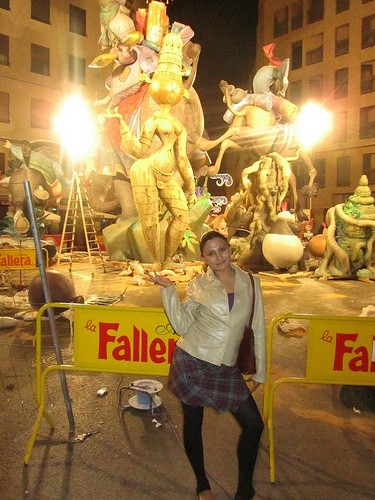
[141, 231, 267, 500]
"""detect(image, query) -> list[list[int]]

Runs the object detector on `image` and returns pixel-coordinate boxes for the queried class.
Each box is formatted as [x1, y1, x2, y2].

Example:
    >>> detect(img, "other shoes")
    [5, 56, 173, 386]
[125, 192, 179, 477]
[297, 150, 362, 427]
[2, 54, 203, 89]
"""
[235, 493, 243, 500]
[196, 487, 215, 500]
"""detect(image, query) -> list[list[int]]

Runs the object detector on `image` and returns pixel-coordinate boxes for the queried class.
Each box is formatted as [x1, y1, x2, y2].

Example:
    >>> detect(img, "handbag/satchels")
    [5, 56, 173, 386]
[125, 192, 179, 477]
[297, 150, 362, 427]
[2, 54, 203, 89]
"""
[237, 328, 256, 374]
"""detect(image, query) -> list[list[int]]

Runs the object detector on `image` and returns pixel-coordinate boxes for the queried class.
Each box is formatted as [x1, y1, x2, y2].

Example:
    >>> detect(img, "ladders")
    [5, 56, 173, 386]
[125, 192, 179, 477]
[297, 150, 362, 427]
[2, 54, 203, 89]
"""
[56, 173, 107, 277]
